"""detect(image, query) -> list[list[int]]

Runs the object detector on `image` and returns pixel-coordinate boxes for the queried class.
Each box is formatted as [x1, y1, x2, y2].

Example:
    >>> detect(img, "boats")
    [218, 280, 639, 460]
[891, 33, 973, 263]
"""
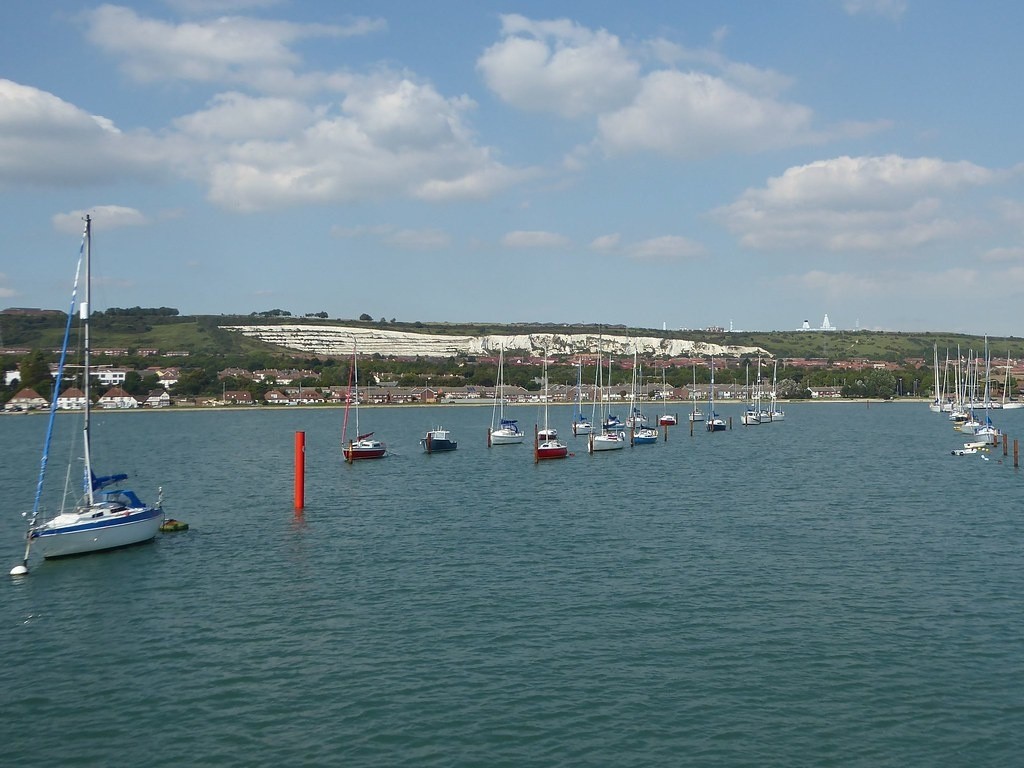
[421, 426, 459, 452]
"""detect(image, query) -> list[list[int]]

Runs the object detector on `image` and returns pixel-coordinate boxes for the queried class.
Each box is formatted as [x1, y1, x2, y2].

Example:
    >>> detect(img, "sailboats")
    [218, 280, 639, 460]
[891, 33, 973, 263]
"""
[689, 361, 704, 421]
[19, 213, 166, 560]
[532, 341, 569, 458]
[489, 341, 525, 444]
[572, 353, 596, 439]
[928, 332, 1024, 443]
[621, 348, 676, 444]
[603, 353, 626, 432]
[339, 337, 388, 462]
[537, 356, 557, 440]
[587, 338, 628, 453]
[707, 355, 726, 431]
[740, 354, 785, 425]
